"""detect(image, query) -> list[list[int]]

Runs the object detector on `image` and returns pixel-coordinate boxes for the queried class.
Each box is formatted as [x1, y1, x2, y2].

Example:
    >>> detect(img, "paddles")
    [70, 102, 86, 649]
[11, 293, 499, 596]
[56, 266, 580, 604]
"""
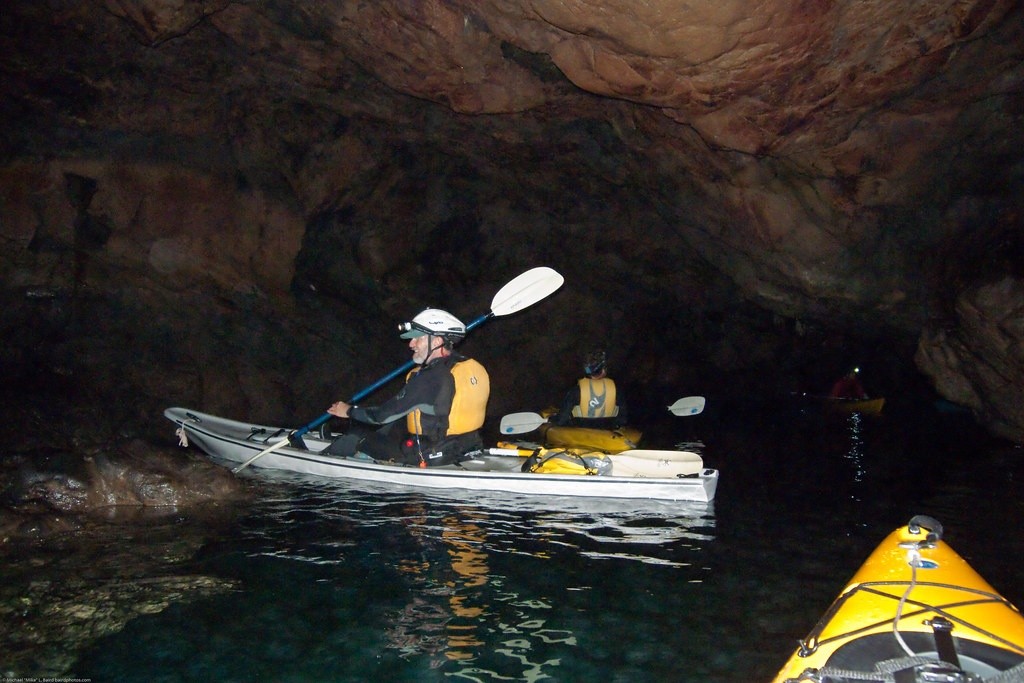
[500, 395, 706, 435]
[232, 261, 566, 476]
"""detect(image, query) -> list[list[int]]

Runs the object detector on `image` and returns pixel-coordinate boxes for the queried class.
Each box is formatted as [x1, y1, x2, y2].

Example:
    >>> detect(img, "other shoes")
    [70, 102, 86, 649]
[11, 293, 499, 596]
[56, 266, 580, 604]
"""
[288, 429, 309, 451]
[320, 422, 330, 440]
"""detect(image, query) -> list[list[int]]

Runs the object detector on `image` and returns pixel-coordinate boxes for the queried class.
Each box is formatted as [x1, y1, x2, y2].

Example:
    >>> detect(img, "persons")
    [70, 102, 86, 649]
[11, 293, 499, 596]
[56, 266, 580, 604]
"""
[550, 349, 618, 423]
[328, 309, 492, 467]
[829, 367, 863, 400]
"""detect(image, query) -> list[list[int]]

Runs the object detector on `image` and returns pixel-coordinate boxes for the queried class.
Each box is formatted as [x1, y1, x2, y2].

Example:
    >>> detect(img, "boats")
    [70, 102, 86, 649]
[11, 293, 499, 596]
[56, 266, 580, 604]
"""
[164, 408, 719, 502]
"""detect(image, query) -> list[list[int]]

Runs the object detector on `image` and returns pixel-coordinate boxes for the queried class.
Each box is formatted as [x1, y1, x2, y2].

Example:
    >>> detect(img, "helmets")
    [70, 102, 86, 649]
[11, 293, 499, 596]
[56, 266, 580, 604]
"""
[411, 309, 465, 335]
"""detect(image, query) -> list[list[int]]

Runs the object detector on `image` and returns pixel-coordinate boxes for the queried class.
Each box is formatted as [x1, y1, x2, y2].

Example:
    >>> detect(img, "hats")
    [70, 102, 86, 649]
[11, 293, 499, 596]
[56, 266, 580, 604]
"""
[399, 329, 426, 339]
[583, 349, 609, 375]
